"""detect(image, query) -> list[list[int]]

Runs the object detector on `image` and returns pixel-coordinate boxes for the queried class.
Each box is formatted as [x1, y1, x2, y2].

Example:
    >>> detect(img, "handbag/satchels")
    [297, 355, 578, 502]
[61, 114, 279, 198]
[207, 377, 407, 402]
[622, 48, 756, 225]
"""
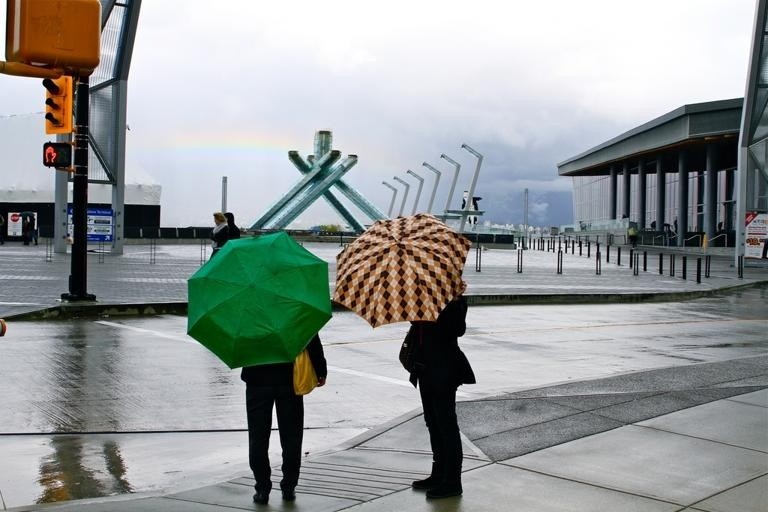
[293, 351, 319, 395]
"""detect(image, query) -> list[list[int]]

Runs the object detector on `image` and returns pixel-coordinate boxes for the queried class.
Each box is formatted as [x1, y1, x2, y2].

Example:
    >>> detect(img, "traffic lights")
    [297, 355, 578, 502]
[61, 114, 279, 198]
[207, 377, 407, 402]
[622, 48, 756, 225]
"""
[42, 75, 72, 134]
[43, 142, 72, 168]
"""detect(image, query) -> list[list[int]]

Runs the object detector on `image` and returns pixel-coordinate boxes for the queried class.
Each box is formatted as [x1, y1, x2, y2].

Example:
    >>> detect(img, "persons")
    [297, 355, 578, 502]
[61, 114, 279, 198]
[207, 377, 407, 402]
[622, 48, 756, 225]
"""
[0, 213, 5, 245]
[207, 211, 227, 259]
[241, 331, 326, 502]
[26, 215, 38, 245]
[223, 212, 240, 243]
[407, 290, 477, 497]
[21, 215, 30, 245]
[626, 222, 639, 250]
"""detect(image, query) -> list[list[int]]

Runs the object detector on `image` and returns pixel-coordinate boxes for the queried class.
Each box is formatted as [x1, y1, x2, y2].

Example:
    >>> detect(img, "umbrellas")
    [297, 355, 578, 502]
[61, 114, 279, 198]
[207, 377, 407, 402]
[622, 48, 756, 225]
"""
[329, 214, 470, 327]
[18, 211, 32, 216]
[186, 227, 331, 372]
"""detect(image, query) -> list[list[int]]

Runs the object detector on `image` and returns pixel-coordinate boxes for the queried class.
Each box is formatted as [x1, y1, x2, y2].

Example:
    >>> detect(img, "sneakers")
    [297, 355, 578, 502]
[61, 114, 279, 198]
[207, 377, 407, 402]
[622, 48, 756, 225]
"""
[282, 488, 296, 501]
[253, 482, 271, 504]
[412, 462, 462, 498]
